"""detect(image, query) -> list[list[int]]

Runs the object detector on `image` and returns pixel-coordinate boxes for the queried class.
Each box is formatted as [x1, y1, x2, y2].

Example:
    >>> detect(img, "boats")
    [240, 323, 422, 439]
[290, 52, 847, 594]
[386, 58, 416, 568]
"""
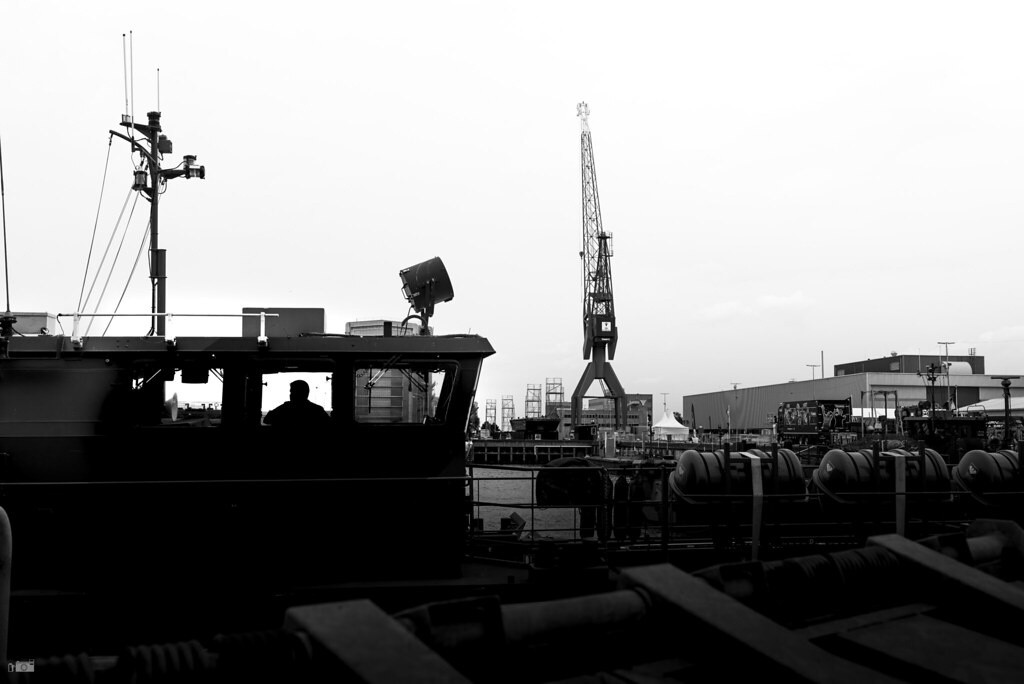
[0, 113, 498, 545]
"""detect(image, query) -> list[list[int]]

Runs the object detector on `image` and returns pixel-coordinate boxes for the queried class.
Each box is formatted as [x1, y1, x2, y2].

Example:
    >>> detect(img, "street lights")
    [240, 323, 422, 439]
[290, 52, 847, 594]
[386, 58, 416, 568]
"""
[915, 363, 952, 432]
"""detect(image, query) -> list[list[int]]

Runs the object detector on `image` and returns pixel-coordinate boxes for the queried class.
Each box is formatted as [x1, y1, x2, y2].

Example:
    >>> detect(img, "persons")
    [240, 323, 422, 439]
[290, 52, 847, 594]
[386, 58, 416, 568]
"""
[262, 380, 331, 426]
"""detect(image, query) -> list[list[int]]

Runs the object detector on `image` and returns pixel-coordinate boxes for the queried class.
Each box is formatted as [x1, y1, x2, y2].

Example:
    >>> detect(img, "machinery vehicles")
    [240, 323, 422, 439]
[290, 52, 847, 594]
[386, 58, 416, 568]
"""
[559, 130, 632, 441]
